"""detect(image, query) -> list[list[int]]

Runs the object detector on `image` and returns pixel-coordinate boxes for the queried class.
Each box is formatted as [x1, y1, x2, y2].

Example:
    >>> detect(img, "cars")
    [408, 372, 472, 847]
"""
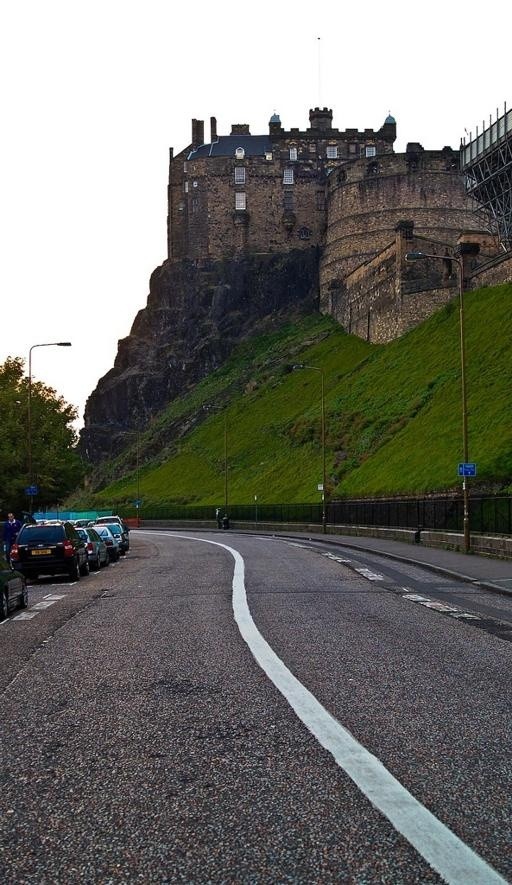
[75, 525, 111, 572]
[0, 551, 30, 622]
[91, 527, 121, 563]
[74, 519, 92, 526]
[95, 523, 128, 557]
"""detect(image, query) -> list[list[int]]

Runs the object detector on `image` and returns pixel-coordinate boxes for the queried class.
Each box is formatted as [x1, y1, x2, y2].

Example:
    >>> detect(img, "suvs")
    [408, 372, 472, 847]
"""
[11, 520, 90, 582]
[96, 515, 127, 531]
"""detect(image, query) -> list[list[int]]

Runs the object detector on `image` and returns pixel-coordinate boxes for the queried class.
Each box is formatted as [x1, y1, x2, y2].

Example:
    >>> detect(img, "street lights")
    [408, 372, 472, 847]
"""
[201, 403, 230, 520]
[292, 364, 327, 533]
[120, 430, 143, 530]
[26, 341, 75, 518]
[403, 249, 475, 551]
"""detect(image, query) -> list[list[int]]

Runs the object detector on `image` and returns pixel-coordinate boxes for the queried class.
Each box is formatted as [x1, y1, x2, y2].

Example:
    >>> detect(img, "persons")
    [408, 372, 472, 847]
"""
[2, 512, 22, 563]
